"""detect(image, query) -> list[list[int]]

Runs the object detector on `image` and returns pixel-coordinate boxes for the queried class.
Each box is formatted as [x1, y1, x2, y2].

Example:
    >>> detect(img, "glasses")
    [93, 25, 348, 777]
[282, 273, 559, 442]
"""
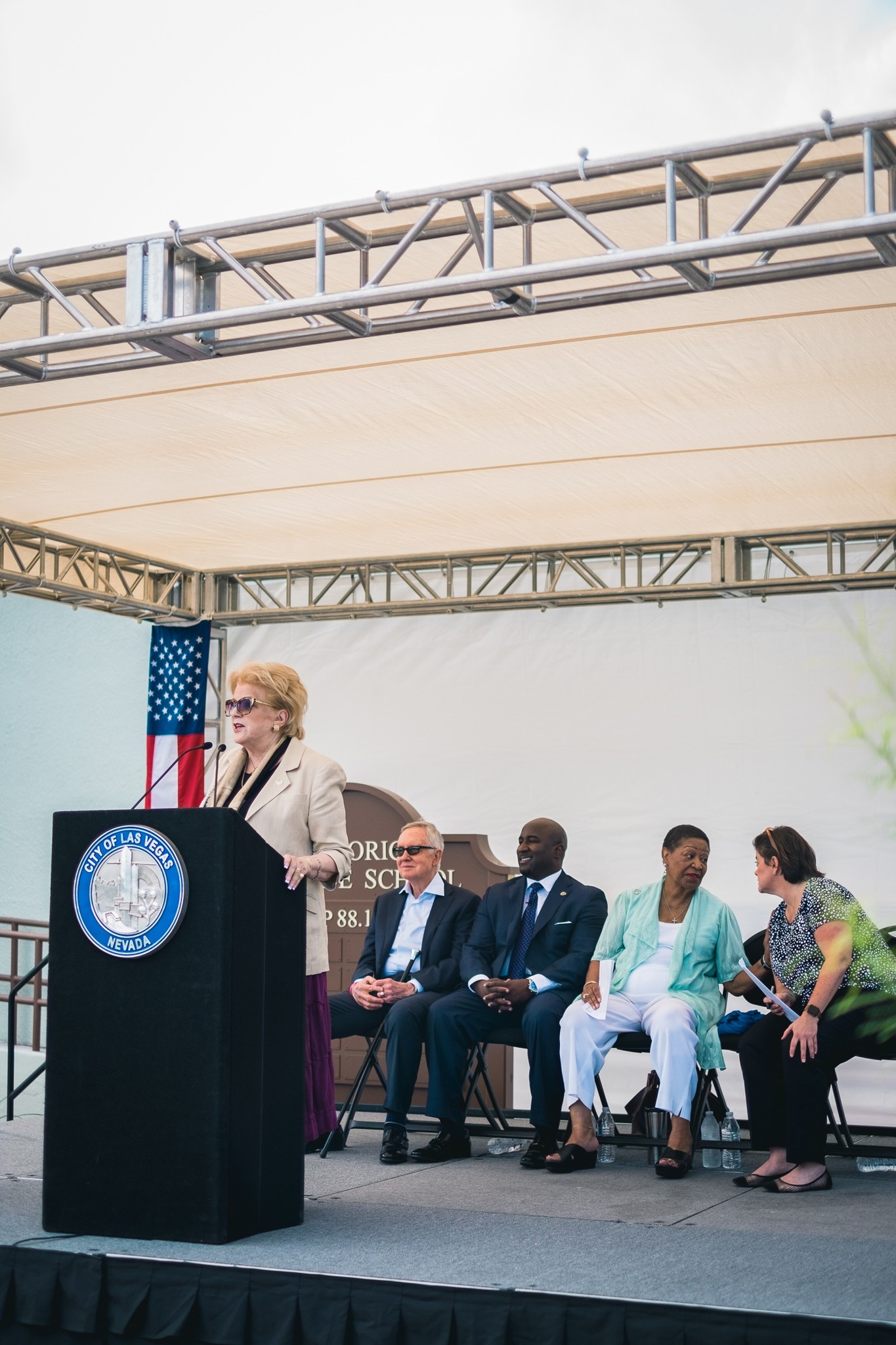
[392, 845, 433, 857]
[224, 697, 280, 718]
[765, 827, 780, 857]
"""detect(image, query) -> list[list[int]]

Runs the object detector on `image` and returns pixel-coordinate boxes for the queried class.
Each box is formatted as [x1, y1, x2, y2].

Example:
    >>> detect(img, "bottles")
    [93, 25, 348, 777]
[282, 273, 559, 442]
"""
[595, 1107, 615, 1163]
[487, 1137, 527, 1155]
[701, 1111, 721, 1168]
[722, 1112, 741, 1169]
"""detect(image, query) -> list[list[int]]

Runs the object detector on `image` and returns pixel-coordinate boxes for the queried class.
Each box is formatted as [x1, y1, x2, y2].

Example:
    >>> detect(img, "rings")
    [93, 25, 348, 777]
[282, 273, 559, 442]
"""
[583, 993, 589, 999]
[381, 992, 384, 998]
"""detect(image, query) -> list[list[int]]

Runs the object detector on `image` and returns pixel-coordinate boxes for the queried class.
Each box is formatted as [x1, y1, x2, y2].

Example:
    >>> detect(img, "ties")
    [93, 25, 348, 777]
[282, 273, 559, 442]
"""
[508, 883, 544, 980]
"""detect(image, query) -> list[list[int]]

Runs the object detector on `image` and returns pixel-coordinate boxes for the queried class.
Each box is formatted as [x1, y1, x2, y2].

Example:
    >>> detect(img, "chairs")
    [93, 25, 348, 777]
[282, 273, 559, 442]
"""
[319, 922, 896, 1160]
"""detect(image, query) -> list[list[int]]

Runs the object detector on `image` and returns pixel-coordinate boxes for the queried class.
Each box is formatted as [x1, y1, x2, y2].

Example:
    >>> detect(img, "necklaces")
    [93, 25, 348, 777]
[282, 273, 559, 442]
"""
[787, 884, 798, 922]
[249, 757, 258, 774]
[662, 880, 693, 923]
[241, 758, 248, 788]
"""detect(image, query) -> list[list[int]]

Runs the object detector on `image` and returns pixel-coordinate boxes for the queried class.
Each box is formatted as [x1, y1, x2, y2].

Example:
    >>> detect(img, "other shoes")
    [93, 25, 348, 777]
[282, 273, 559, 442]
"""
[760, 1170, 833, 1192]
[732, 1164, 800, 1187]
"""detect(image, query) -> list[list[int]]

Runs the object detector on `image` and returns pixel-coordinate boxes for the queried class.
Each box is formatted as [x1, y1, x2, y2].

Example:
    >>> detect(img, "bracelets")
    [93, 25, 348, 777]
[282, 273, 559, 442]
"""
[349, 982, 357, 996]
[302, 857, 312, 877]
[311, 855, 323, 881]
[760, 954, 771, 970]
[583, 981, 597, 991]
[781, 991, 796, 1000]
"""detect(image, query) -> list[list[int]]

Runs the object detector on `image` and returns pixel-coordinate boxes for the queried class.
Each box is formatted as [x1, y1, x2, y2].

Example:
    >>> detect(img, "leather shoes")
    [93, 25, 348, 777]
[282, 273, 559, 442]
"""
[379, 1128, 409, 1164]
[520, 1141, 560, 1169]
[410, 1129, 472, 1161]
[305, 1124, 345, 1151]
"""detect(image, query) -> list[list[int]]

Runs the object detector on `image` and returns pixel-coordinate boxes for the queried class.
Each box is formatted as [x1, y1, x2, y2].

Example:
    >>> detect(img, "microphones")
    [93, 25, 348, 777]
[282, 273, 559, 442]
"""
[131, 742, 213, 809]
[214, 742, 228, 807]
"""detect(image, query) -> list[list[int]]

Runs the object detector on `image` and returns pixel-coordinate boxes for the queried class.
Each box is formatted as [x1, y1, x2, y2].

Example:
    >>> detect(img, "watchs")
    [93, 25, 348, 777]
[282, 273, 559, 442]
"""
[804, 1004, 822, 1021]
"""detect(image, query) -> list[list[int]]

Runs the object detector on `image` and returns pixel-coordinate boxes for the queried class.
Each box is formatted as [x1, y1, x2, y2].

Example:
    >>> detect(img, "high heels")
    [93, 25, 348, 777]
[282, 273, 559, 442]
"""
[544, 1144, 598, 1173]
[655, 1145, 695, 1179]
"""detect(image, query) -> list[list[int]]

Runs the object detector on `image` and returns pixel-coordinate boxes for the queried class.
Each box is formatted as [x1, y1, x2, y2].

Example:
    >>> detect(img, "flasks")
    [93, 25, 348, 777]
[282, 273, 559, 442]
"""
[645, 1107, 668, 1165]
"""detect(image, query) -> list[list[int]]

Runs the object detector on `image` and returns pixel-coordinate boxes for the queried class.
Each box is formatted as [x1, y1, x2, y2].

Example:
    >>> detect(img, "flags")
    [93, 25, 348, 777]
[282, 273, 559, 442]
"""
[145, 616, 214, 809]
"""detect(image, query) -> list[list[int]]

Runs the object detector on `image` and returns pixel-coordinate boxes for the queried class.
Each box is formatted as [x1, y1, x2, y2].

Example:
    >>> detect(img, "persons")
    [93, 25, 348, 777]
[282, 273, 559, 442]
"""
[731, 826, 896, 1192]
[200, 664, 354, 1153]
[305, 822, 482, 1164]
[545, 826, 777, 1180]
[408, 818, 608, 1169]
